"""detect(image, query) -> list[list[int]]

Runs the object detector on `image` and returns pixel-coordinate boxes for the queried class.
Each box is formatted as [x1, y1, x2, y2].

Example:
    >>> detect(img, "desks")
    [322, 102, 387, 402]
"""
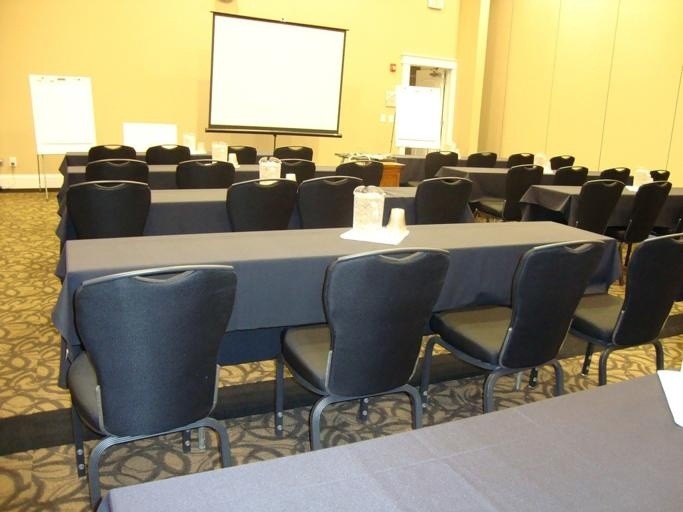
[51, 221, 621, 481]
[96, 368, 683, 508]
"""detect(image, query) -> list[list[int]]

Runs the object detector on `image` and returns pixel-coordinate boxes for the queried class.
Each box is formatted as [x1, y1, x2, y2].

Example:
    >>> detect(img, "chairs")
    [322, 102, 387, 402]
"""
[422, 237, 605, 416]
[272, 248, 453, 453]
[51, 145, 683, 288]
[51, 144, 682, 510]
[526, 233, 682, 390]
[67, 262, 239, 508]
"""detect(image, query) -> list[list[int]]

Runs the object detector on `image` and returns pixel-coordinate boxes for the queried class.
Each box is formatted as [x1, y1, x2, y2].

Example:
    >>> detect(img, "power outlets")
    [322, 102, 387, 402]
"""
[8, 157, 16, 167]
